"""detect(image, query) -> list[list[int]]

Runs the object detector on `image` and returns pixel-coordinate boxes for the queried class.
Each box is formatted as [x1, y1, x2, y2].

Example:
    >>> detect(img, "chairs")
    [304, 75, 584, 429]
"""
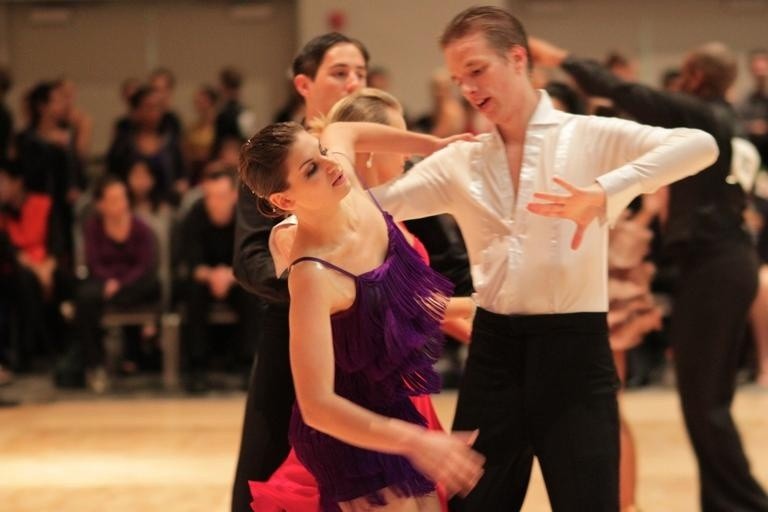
[71, 141, 243, 390]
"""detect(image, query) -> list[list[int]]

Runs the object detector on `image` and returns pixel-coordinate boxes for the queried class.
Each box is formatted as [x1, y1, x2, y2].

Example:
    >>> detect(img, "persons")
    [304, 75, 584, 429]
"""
[527, 33, 768, 512]
[228, 5, 719, 512]
[2, 46, 768, 394]
